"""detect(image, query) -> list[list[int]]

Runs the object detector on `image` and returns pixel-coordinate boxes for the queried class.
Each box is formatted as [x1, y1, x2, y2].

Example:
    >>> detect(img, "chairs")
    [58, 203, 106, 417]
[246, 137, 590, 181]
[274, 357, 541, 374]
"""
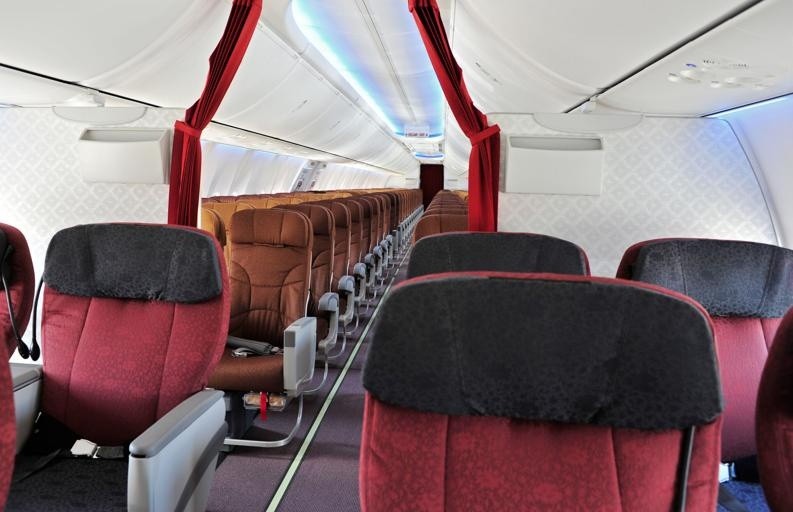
[200, 207, 227, 254]
[274, 205, 341, 395]
[0, 220, 37, 364]
[236, 198, 267, 209]
[364, 196, 385, 287]
[213, 196, 235, 203]
[266, 198, 302, 208]
[221, 208, 318, 453]
[236, 195, 272, 202]
[201, 197, 218, 203]
[296, 200, 358, 334]
[358, 272, 724, 512]
[9, 223, 230, 512]
[329, 197, 369, 315]
[406, 232, 591, 279]
[616, 239, 793, 512]
[202, 202, 256, 268]
[755, 305, 793, 512]
[266, 189, 469, 237]
[0, 319, 16, 511]
